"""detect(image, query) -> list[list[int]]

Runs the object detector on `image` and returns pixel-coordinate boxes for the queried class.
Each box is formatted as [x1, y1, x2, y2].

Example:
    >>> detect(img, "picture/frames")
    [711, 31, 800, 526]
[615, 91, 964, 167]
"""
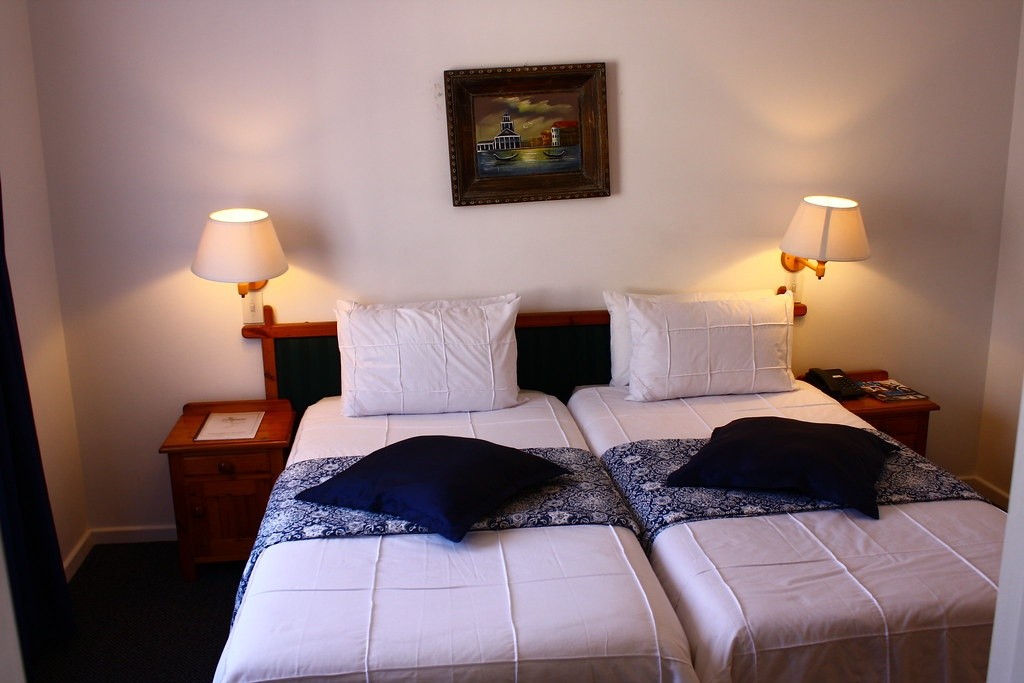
[444, 62, 611, 207]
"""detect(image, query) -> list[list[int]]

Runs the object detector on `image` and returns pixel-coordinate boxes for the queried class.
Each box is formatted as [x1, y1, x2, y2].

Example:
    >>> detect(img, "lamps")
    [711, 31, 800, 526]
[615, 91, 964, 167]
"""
[782, 195, 871, 281]
[189, 208, 291, 323]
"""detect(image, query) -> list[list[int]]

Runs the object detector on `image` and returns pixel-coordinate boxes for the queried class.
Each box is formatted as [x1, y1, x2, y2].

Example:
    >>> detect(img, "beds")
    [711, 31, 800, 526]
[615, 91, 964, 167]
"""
[571, 302, 1009, 683]
[209, 304, 701, 683]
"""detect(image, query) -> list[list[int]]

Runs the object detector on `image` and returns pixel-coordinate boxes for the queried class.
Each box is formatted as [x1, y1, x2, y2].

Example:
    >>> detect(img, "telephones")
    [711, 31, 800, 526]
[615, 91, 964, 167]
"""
[805, 367, 866, 399]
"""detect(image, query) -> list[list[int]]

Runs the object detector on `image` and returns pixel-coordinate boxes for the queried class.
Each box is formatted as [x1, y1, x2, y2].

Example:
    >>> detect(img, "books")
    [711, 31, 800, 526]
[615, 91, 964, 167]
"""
[193, 412, 265, 440]
[858, 380, 928, 403]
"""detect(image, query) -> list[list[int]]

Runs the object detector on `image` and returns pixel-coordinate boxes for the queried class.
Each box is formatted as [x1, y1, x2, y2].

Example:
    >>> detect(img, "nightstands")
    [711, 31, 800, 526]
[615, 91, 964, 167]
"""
[799, 371, 941, 458]
[157, 398, 297, 575]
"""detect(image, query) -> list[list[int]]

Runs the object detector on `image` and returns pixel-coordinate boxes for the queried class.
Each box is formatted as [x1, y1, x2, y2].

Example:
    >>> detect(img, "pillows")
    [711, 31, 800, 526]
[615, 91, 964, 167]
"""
[293, 435, 574, 543]
[668, 414, 903, 520]
[622, 290, 804, 402]
[603, 288, 776, 388]
[333, 291, 531, 415]
